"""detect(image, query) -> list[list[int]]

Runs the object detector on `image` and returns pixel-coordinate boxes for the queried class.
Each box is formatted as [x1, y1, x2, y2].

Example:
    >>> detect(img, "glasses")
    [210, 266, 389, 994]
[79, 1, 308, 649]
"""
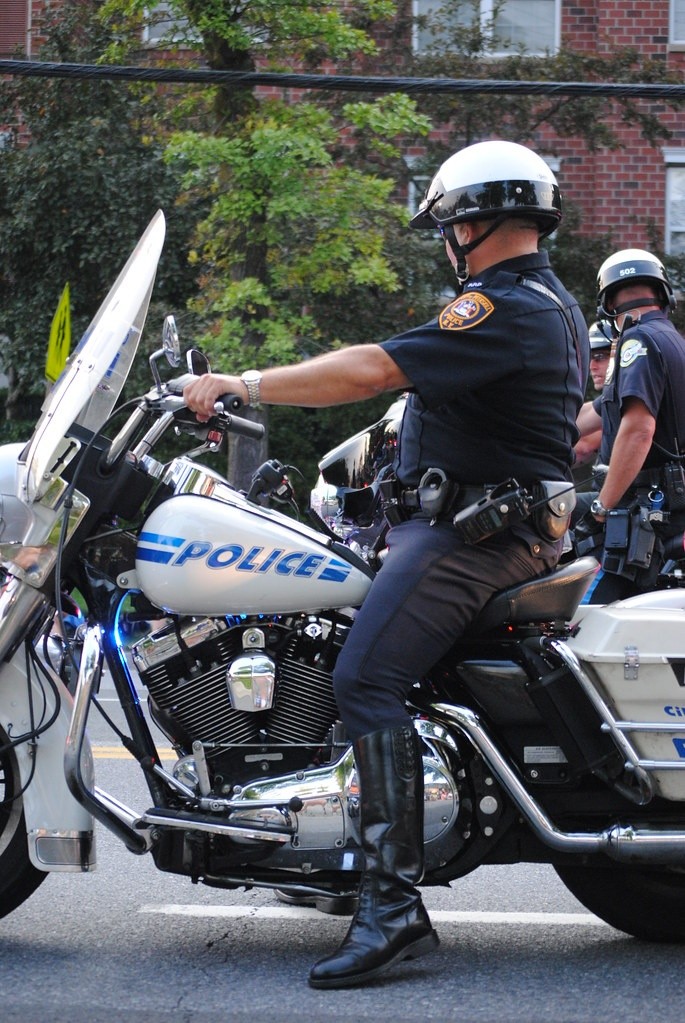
[589, 351, 611, 359]
[437, 224, 446, 241]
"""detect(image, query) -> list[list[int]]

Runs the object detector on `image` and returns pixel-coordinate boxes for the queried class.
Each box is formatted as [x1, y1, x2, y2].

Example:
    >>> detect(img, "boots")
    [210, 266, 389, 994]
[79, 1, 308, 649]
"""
[307, 726, 439, 988]
[273, 888, 357, 916]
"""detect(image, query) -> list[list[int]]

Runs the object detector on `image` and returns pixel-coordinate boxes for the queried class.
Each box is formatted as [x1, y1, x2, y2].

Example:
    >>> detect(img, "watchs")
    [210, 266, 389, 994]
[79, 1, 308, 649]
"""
[240, 368, 263, 408]
[590, 500, 608, 516]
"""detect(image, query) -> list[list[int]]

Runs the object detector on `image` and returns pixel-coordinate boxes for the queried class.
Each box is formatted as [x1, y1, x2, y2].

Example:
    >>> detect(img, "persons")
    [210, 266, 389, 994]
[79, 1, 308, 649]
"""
[571, 249, 685, 605]
[183, 140, 590, 990]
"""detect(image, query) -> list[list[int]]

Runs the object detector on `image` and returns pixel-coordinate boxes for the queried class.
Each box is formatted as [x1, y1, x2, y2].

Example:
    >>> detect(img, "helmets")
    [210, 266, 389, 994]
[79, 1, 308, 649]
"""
[588, 319, 614, 349]
[595, 248, 675, 295]
[408, 141, 563, 228]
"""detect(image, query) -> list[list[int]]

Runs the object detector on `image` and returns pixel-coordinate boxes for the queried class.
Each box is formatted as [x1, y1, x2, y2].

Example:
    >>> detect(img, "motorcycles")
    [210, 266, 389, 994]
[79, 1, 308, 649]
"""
[0, 207, 684, 947]
